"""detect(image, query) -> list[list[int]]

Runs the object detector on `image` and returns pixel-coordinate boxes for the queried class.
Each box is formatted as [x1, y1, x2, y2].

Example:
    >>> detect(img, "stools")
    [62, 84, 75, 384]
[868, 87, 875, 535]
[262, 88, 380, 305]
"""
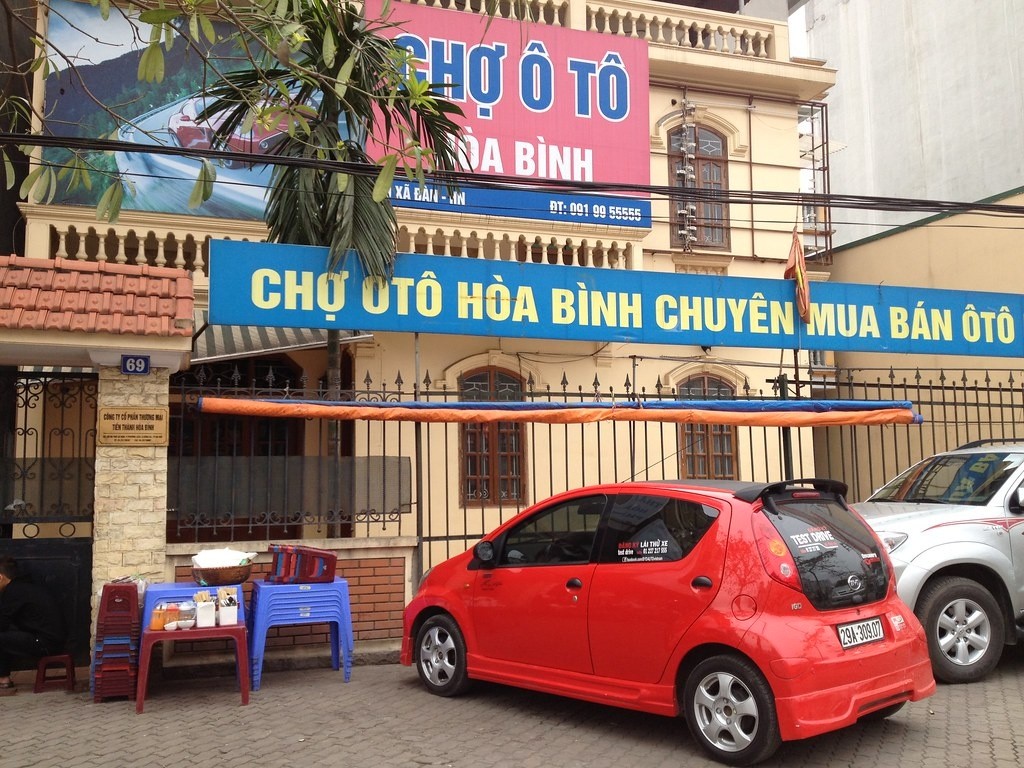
[33, 654, 76, 692]
[263, 542, 339, 585]
[88, 582, 141, 716]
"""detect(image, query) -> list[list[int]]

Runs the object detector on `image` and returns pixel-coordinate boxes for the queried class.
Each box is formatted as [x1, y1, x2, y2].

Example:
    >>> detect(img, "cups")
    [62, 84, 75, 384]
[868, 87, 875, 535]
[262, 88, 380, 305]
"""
[179, 606, 196, 620]
[148, 611, 164, 630]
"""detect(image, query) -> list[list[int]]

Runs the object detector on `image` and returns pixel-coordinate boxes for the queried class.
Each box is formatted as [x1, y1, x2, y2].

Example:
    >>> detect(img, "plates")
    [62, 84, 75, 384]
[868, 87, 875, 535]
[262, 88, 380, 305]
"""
[164, 622, 176, 631]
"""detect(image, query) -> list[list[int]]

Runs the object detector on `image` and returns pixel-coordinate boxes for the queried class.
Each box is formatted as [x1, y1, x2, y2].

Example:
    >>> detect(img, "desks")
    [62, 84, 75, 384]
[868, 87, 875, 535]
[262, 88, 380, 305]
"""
[135, 584, 249, 715]
[249, 577, 355, 690]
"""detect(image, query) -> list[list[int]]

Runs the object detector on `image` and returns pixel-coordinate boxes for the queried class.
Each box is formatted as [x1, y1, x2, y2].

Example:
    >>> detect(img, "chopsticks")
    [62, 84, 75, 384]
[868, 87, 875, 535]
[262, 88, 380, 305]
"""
[217, 587, 237, 600]
[194, 590, 210, 602]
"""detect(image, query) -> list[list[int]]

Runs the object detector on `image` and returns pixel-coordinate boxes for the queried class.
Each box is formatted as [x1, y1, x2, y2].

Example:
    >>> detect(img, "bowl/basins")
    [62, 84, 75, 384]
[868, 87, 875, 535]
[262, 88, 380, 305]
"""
[192, 562, 252, 586]
[177, 620, 195, 630]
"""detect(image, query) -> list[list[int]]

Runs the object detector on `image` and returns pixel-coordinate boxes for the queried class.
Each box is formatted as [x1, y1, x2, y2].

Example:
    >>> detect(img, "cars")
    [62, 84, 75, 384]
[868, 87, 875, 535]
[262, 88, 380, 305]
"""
[167, 91, 313, 170]
[400, 477, 938, 768]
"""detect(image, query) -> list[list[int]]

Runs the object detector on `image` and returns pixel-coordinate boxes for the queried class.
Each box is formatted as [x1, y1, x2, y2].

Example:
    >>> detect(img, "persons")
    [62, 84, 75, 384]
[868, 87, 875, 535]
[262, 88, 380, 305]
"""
[0, 559, 68, 688]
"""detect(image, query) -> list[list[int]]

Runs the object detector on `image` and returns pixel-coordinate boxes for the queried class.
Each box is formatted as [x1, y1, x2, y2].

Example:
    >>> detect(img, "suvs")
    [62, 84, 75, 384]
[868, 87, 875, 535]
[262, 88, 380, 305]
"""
[850, 438, 1024, 684]
